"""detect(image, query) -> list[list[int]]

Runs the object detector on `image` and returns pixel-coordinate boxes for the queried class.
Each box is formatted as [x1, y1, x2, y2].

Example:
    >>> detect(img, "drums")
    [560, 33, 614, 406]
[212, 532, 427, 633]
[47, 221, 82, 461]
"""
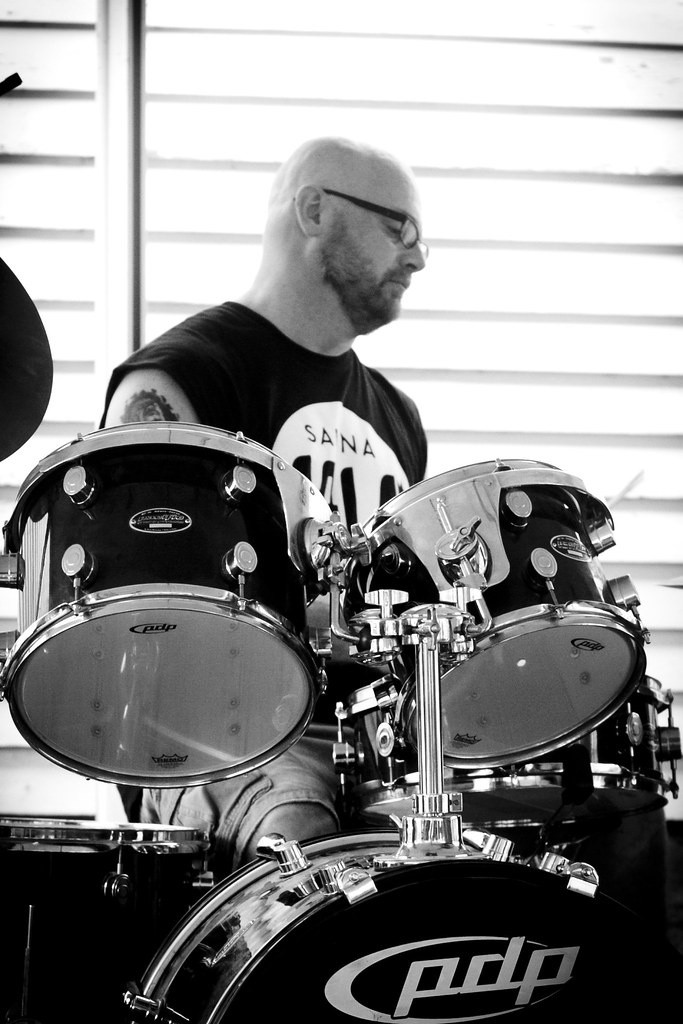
[119, 822, 683, 1024]
[358, 673, 669, 833]
[0, 811, 216, 1024]
[0, 418, 346, 791]
[337, 457, 647, 771]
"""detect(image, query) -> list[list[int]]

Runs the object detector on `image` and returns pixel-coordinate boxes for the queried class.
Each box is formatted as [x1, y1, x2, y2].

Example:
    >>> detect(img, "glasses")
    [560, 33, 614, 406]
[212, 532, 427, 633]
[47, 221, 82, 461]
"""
[323, 189, 429, 260]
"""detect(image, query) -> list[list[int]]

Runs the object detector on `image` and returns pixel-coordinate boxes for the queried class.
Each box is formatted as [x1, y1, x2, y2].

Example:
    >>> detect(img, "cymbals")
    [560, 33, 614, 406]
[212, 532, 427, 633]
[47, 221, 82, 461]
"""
[0, 253, 56, 463]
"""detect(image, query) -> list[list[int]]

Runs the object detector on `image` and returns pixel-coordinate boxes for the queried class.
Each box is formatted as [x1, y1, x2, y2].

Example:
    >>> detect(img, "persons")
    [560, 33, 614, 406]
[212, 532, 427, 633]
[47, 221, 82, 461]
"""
[95, 136, 558, 871]
